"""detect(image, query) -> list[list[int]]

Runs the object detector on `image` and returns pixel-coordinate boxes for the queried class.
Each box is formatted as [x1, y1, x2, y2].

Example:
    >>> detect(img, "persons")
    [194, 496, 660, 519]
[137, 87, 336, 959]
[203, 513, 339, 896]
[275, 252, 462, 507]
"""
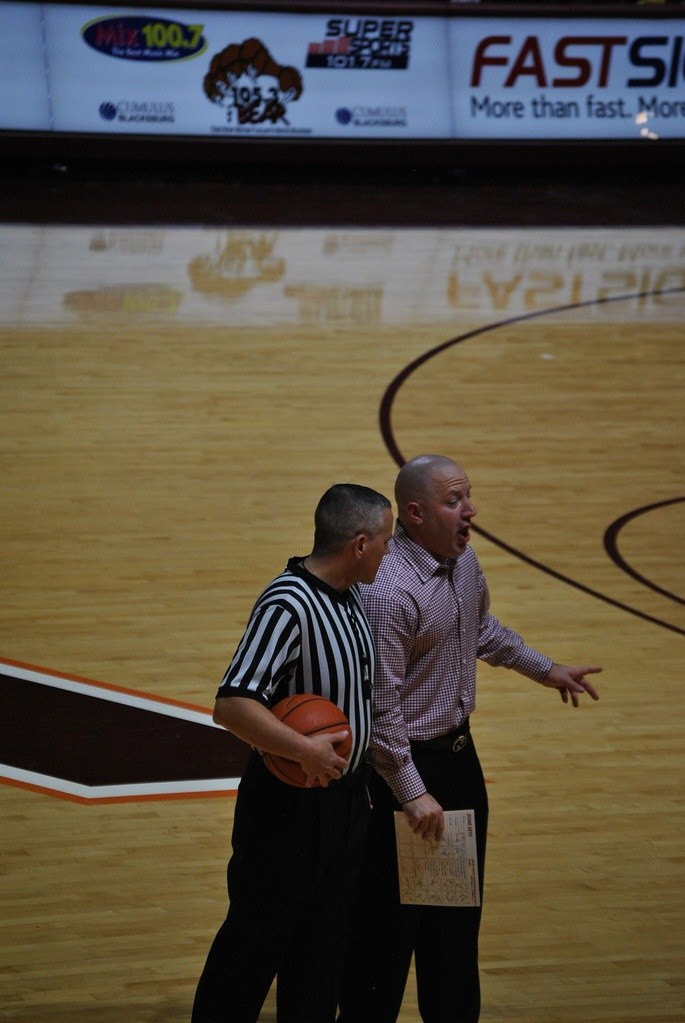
[357, 454, 604, 1023]
[191, 483, 394, 1023]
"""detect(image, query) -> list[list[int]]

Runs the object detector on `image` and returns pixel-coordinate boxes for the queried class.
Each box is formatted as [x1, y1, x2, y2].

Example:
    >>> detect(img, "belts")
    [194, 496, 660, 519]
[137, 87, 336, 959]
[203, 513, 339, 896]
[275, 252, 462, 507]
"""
[414, 724, 472, 758]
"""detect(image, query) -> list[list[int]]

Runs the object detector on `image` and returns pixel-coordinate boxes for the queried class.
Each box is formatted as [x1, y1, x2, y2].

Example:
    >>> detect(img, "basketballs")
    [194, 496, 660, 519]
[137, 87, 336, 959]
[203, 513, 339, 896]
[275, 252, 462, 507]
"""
[262, 692, 355, 790]
